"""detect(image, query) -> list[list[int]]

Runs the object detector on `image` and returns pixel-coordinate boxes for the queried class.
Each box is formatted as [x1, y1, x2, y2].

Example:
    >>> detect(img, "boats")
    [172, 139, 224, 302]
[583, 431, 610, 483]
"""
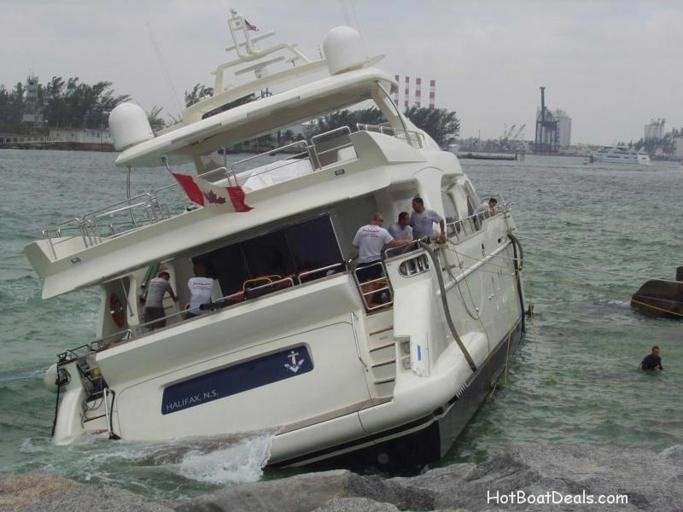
[596, 143, 652, 164]
[628, 264, 683, 323]
[20, 6, 535, 480]
[583, 153, 593, 165]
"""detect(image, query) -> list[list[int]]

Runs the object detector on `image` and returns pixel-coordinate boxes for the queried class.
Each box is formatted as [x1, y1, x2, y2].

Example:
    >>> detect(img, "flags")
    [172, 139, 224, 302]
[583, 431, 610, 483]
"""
[172, 170, 257, 214]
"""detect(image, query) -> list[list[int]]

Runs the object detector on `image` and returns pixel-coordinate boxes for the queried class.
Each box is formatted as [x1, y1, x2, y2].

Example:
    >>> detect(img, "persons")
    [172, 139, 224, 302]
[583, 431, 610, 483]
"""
[144, 270, 179, 331]
[410, 197, 446, 244]
[388, 210, 414, 252]
[184, 264, 214, 322]
[473, 198, 496, 225]
[640, 346, 664, 372]
[351, 210, 414, 307]
